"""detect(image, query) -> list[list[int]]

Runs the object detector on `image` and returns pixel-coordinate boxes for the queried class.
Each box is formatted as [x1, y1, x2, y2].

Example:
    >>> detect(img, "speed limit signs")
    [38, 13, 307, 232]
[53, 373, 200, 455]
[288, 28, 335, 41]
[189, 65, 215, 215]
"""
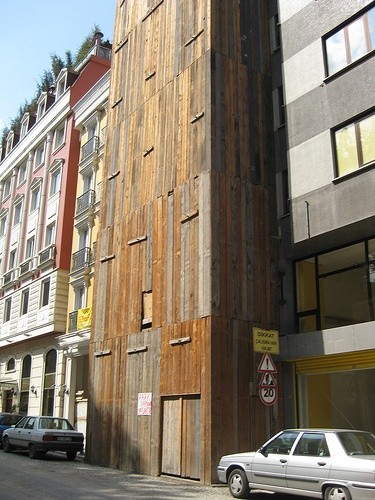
[258, 387, 277, 406]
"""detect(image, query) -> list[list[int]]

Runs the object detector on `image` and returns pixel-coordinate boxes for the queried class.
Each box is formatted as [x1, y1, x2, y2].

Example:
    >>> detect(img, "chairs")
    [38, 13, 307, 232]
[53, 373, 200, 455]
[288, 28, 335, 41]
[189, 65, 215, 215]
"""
[49, 423, 56, 428]
[306, 442, 318, 455]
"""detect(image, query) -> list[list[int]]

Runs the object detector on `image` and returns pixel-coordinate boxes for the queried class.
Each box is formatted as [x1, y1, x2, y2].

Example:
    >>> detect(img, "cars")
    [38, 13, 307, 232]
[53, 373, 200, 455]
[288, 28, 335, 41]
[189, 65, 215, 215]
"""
[217, 427, 375, 500]
[3, 416, 84, 462]
[0, 413, 23, 450]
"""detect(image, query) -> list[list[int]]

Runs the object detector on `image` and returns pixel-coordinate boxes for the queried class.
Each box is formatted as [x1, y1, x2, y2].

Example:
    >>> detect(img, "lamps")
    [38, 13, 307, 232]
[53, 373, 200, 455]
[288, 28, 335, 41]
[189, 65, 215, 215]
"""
[11, 387, 15, 393]
[31, 385, 34, 392]
